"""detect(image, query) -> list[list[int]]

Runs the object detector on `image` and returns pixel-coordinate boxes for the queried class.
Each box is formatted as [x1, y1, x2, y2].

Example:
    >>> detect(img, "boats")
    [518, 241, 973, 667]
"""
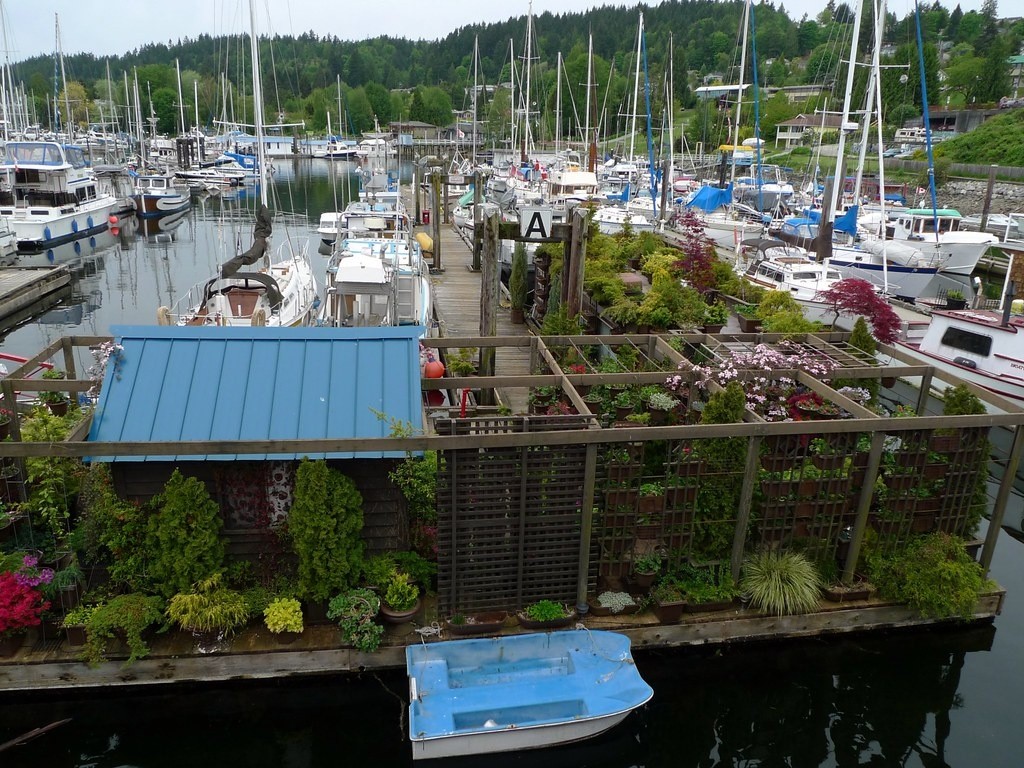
[406, 631, 654, 759]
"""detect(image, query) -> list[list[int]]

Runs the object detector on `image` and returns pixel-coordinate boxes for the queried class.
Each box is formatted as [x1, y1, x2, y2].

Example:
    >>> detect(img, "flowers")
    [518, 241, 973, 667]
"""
[795, 399, 820, 411]
[0, 570, 52, 638]
[818, 404, 839, 415]
[872, 404, 891, 417]
[662, 337, 840, 424]
[15, 553, 55, 586]
[837, 387, 871, 401]
[883, 436, 902, 451]
[562, 364, 588, 375]
[546, 401, 571, 415]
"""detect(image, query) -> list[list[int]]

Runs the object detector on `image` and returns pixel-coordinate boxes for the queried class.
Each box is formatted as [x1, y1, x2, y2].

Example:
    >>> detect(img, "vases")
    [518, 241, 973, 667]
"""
[0, 631, 20, 656]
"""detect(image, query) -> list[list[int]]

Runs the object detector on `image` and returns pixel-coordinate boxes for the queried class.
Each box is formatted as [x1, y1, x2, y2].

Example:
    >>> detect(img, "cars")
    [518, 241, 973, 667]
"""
[882, 148, 903, 159]
[894, 149, 915, 160]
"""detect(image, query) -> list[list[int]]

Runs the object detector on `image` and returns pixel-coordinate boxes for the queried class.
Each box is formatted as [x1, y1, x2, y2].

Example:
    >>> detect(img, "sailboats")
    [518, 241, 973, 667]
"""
[0, 0, 1024, 536]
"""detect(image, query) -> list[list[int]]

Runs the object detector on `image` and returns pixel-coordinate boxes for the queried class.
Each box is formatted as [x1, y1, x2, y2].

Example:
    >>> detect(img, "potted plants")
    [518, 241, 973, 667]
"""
[587, 591, 639, 617]
[444, 610, 508, 635]
[680, 562, 743, 612]
[529, 199, 963, 576]
[823, 581, 871, 602]
[650, 575, 687, 625]
[508, 243, 528, 324]
[0, 370, 432, 668]
[516, 600, 576, 628]
